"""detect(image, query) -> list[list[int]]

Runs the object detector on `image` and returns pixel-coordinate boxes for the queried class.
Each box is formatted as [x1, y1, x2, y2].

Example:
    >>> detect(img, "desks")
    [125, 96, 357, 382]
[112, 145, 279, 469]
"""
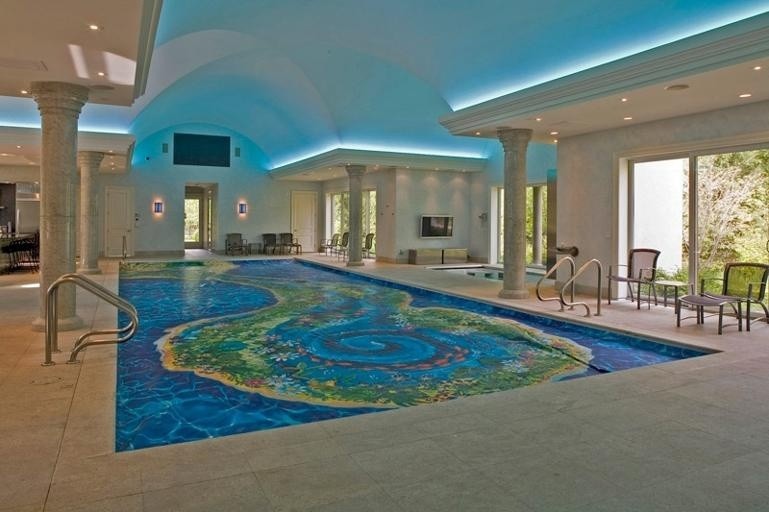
[648, 280, 687, 315]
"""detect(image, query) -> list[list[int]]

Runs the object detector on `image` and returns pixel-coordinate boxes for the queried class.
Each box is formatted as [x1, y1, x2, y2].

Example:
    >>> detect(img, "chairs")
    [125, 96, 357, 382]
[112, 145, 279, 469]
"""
[2, 230, 40, 274]
[606, 249, 661, 310]
[700, 261, 769, 331]
[225, 233, 302, 257]
[318, 232, 374, 262]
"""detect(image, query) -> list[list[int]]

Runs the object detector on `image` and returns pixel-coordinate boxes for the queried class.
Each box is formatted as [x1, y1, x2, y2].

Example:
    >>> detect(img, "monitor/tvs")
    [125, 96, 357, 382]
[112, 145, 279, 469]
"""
[420, 214, 456, 239]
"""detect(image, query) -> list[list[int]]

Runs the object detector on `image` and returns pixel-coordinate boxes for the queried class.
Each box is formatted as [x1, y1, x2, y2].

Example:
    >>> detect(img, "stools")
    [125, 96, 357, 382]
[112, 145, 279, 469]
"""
[676, 294, 741, 335]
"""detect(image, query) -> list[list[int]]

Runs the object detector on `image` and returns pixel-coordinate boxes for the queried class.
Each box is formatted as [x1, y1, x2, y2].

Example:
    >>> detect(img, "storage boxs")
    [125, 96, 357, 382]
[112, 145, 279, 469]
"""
[409, 248, 442, 264]
[443, 248, 468, 263]
